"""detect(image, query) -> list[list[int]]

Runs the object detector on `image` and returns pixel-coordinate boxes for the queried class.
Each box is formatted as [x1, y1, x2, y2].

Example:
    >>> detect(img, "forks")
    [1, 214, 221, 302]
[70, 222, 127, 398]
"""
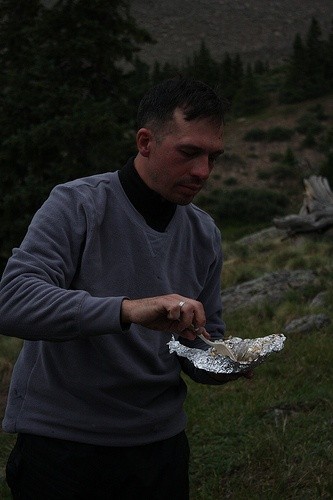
[177, 317, 236, 361]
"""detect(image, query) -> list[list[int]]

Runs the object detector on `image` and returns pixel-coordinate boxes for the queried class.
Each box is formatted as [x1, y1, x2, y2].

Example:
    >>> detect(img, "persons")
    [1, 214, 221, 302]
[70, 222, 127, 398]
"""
[0, 76, 255, 500]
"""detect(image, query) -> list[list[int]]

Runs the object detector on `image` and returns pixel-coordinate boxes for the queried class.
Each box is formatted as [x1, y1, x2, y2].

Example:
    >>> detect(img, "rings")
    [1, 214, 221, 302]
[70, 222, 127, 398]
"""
[178, 300, 186, 309]
